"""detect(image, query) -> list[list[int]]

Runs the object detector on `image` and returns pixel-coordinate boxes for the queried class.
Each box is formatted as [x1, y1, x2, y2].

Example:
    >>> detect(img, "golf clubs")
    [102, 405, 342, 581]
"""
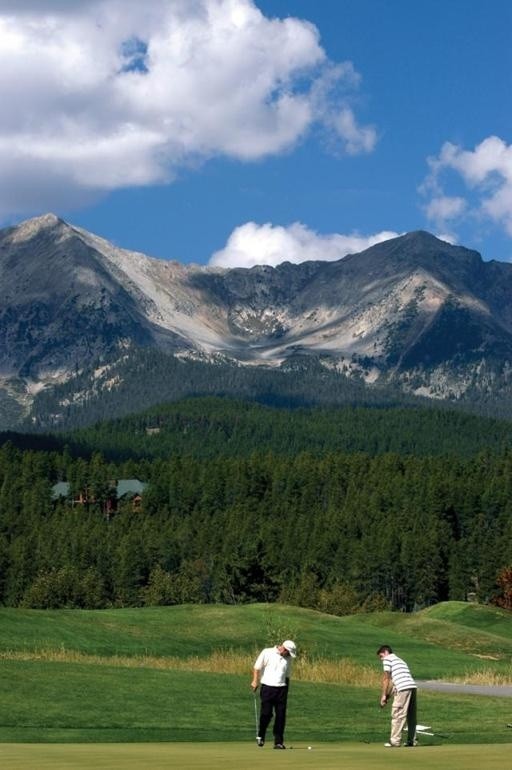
[254, 688, 261, 740]
[362, 706, 383, 744]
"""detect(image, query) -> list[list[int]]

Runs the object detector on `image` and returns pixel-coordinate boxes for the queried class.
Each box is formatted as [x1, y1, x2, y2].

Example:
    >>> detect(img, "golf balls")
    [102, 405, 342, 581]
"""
[308, 746, 313, 750]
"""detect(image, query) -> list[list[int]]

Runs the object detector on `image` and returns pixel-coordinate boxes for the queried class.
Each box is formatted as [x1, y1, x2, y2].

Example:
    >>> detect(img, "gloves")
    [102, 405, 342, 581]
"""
[380, 696, 387, 706]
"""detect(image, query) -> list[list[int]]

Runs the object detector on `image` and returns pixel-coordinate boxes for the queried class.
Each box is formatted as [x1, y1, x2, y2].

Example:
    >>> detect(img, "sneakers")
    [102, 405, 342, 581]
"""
[258, 739, 286, 749]
[384, 741, 417, 747]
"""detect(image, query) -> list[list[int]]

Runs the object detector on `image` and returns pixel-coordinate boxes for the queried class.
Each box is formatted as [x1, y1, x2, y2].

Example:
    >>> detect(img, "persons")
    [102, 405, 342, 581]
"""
[251, 639, 298, 749]
[376, 644, 419, 747]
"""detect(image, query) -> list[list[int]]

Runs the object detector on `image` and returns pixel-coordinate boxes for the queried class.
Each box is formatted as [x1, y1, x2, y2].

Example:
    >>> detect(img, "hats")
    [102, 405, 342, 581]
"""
[283, 640, 297, 658]
